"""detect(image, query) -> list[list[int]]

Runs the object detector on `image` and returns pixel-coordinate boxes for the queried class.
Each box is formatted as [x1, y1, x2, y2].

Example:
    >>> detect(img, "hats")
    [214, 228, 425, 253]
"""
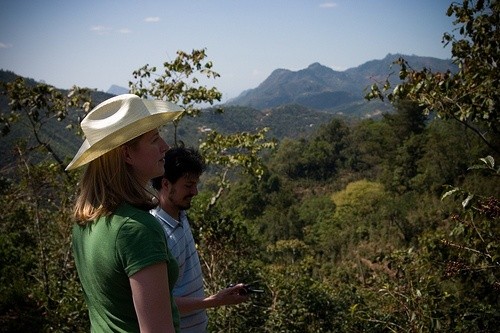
[65, 93, 185, 172]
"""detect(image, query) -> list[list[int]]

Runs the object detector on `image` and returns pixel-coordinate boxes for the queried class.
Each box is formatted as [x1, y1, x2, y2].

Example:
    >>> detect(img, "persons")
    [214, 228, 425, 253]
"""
[149, 141, 250, 333]
[64, 94, 185, 333]
